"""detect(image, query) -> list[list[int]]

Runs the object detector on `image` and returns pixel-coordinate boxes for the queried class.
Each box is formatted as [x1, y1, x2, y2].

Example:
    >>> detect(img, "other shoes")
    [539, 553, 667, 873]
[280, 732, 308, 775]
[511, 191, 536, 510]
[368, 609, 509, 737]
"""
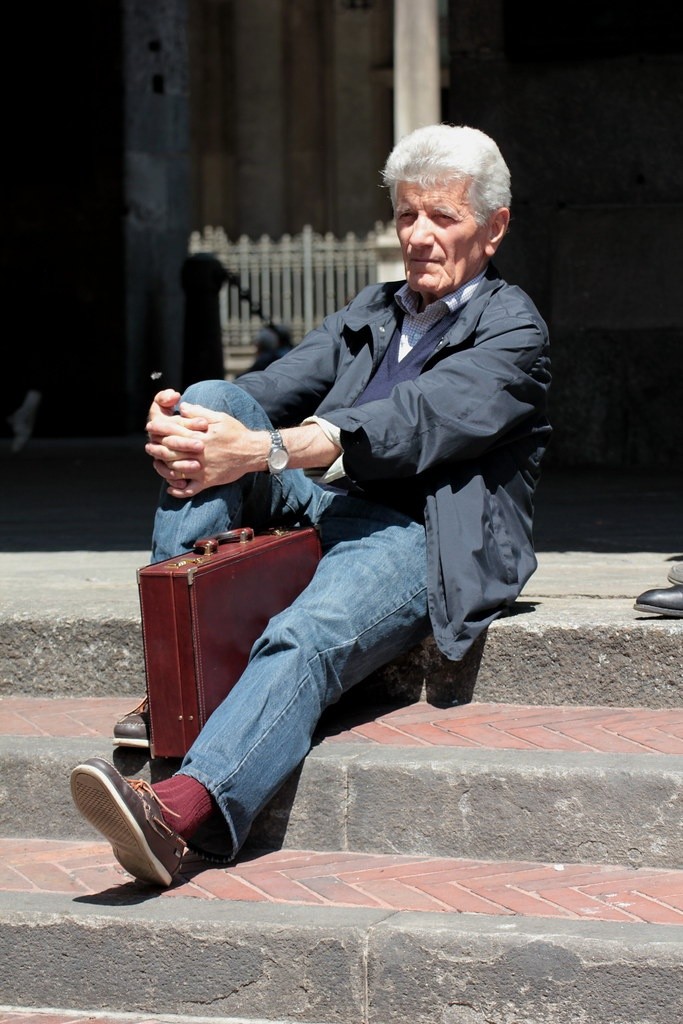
[112, 698, 151, 747]
[70, 757, 188, 887]
[666, 563, 683, 584]
[635, 583, 683, 617]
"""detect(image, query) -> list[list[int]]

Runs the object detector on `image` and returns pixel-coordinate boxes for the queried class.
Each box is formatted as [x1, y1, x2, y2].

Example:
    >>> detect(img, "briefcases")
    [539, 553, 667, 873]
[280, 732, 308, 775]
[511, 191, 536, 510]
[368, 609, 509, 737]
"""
[136, 519, 323, 761]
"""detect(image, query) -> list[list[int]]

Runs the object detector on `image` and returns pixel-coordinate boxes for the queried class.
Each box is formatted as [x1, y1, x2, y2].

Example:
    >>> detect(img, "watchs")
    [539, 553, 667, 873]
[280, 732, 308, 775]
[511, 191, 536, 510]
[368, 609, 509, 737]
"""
[266, 428, 290, 477]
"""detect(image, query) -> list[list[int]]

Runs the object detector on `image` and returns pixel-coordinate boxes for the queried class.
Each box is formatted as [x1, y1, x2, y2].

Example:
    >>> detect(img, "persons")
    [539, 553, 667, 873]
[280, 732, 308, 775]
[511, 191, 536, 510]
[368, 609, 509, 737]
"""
[634, 563, 683, 619]
[69, 127, 552, 889]
